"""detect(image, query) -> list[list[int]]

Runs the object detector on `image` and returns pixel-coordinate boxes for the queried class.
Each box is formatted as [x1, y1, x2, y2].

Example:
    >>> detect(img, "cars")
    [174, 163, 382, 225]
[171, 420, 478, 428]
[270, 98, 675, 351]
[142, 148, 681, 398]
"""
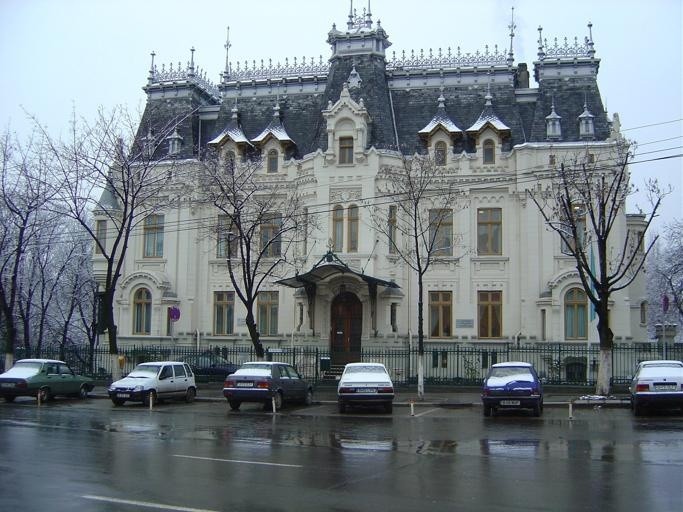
[629, 360, 683, 416]
[336, 362, 395, 413]
[223, 361, 313, 411]
[107, 362, 197, 406]
[481, 362, 544, 417]
[0, 358, 94, 402]
